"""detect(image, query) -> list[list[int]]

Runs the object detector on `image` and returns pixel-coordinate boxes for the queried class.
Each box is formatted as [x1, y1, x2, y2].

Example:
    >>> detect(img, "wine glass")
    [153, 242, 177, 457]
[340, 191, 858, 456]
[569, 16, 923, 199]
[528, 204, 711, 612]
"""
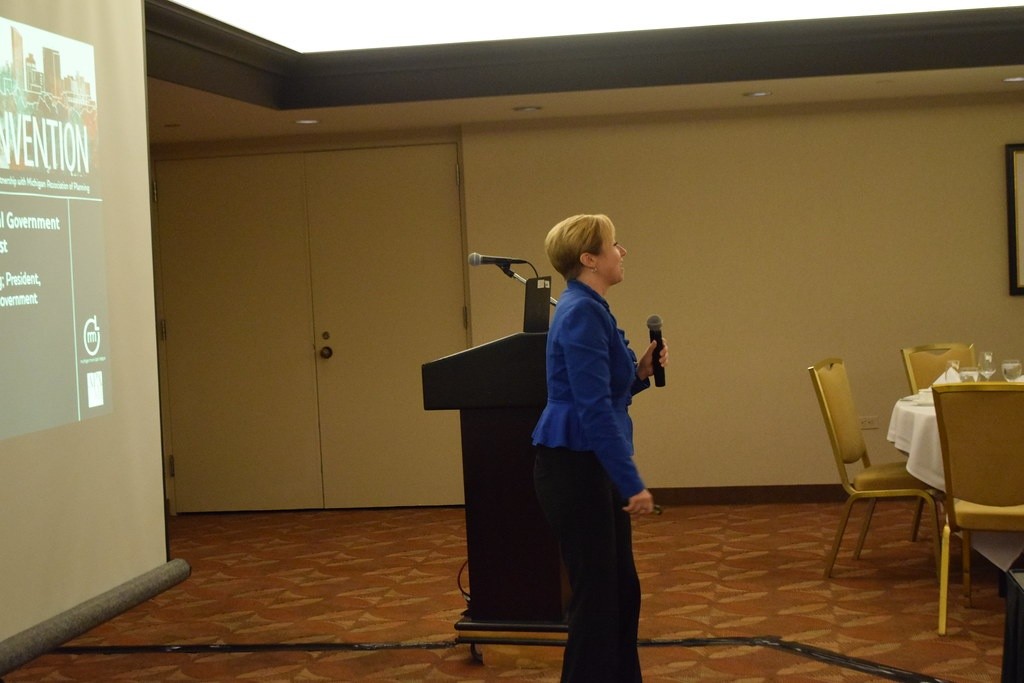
[977, 351, 996, 382]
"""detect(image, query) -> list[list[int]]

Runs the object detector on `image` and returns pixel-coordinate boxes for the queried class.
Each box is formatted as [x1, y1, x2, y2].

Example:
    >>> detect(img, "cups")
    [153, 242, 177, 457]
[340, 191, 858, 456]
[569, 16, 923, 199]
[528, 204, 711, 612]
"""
[1002, 360, 1021, 382]
[919, 389, 933, 400]
[945, 360, 959, 378]
[960, 368, 980, 382]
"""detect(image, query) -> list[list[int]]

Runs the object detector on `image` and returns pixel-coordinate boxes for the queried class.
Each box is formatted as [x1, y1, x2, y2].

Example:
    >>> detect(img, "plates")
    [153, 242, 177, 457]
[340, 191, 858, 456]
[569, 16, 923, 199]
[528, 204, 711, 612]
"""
[912, 400, 935, 405]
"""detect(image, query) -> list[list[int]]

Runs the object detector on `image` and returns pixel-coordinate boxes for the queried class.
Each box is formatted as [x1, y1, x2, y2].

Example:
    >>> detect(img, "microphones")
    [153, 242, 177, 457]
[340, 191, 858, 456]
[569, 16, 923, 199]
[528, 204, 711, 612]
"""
[468, 252, 527, 266]
[647, 314, 665, 388]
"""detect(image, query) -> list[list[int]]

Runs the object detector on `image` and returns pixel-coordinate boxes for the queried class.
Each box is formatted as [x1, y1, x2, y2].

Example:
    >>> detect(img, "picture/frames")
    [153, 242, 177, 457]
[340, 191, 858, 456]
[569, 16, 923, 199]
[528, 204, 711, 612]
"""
[1005, 143, 1024, 296]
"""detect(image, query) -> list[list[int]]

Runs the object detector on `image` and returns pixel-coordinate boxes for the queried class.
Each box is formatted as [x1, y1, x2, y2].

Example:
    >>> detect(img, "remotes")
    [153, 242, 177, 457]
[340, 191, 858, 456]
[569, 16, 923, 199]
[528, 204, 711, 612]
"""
[650, 505, 663, 516]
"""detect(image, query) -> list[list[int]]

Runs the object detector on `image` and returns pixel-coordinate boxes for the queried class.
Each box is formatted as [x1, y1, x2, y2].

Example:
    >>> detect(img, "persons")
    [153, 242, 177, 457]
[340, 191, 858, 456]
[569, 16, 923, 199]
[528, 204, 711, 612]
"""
[533, 214, 669, 683]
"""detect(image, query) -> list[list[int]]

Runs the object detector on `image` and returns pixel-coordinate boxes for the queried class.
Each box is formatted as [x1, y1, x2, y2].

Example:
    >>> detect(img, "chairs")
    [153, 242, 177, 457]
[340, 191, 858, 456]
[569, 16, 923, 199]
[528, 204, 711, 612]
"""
[930, 383, 1024, 636]
[900, 342, 979, 542]
[807, 357, 947, 588]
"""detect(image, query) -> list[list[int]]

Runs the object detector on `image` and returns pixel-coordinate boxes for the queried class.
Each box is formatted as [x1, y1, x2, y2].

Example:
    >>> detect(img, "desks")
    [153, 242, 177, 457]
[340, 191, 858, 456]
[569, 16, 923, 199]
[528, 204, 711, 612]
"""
[895, 395, 944, 493]
